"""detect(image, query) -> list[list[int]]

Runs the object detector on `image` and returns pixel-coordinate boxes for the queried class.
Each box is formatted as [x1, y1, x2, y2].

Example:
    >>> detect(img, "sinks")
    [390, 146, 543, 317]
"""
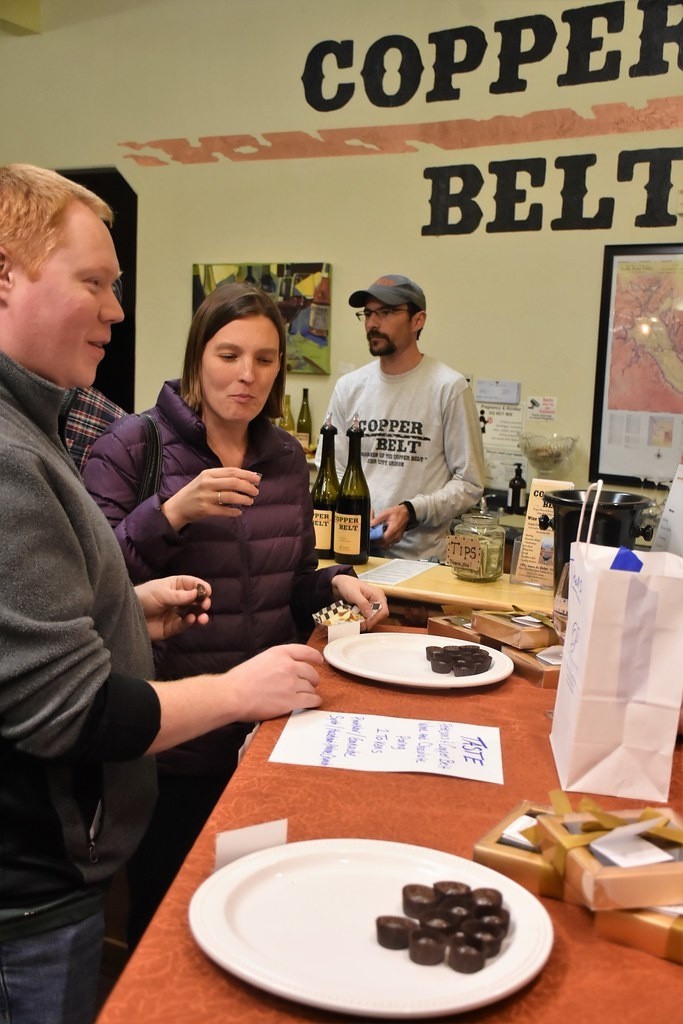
[451, 505, 502, 523]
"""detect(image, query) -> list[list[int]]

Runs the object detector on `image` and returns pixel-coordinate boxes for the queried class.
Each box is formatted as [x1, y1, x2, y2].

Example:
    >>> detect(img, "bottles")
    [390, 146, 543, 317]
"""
[334, 413, 371, 565]
[452, 514, 506, 583]
[309, 263, 329, 337]
[244, 265, 257, 285]
[297, 389, 311, 452]
[192, 264, 207, 321]
[310, 412, 339, 559]
[278, 394, 295, 438]
[279, 263, 291, 302]
[203, 264, 216, 296]
[260, 264, 278, 302]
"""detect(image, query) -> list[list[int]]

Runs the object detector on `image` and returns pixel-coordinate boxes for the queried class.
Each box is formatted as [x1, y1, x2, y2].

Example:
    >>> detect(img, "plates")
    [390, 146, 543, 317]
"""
[322, 631, 513, 687]
[188, 838, 553, 1017]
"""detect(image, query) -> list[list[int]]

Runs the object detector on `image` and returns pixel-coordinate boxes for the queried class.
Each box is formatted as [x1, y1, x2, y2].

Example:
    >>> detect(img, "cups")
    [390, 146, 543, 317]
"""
[554, 563, 569, 641]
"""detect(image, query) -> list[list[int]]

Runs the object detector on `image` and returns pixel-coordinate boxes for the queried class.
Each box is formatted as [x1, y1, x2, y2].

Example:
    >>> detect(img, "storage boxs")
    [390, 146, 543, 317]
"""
[472, 798, 683, 965]
[426, 607, 562, 689]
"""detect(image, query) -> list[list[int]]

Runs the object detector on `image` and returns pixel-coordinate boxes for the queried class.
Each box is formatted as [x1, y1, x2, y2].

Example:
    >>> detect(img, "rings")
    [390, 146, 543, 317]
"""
[217, 492, 223, 506]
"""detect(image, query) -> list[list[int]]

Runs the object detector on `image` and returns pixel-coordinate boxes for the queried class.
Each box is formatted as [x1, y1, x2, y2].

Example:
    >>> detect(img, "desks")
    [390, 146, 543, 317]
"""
[312, 554, 555, 625]
[93, 624, 683, 1024]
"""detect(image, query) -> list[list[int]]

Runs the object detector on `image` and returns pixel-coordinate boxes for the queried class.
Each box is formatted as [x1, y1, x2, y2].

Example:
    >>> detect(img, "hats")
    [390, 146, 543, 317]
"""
[349, 275, 426, 312]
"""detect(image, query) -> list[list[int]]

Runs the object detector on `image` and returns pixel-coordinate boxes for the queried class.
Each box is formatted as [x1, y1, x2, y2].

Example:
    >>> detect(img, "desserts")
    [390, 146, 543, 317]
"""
[377, 882, 510, 973]
[426, 644, 493, 677]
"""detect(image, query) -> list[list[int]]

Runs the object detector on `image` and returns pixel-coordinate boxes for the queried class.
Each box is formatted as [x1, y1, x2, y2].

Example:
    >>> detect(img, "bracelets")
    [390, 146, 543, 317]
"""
[399, 501, 419, 531]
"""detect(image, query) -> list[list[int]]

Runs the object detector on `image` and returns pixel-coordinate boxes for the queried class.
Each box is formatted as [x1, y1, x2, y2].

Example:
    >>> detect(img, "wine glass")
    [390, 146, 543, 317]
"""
[289, 272, 315, 343]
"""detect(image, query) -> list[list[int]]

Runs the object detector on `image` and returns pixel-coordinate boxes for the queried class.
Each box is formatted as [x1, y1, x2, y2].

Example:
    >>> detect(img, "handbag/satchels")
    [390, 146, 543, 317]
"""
[549, 479, 683, 802]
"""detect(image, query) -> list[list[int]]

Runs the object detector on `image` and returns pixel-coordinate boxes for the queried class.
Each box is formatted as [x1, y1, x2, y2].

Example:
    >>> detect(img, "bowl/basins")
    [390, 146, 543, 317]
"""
[518, 432, 583, 474]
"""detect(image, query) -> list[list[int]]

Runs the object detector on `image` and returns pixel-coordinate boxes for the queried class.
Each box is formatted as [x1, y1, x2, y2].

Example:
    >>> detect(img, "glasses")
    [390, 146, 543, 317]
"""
[356, 306, 410, 322]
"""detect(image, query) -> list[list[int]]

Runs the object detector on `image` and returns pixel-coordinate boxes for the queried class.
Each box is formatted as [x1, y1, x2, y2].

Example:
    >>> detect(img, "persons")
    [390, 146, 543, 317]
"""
[313, 275, 486, 563]
[0, 159, 327, 1024]
[78, 278, 390, 948]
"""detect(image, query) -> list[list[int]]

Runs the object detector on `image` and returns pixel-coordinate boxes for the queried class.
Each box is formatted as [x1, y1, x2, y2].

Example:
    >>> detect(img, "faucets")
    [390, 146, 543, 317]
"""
[480, 494, 496, 511]
